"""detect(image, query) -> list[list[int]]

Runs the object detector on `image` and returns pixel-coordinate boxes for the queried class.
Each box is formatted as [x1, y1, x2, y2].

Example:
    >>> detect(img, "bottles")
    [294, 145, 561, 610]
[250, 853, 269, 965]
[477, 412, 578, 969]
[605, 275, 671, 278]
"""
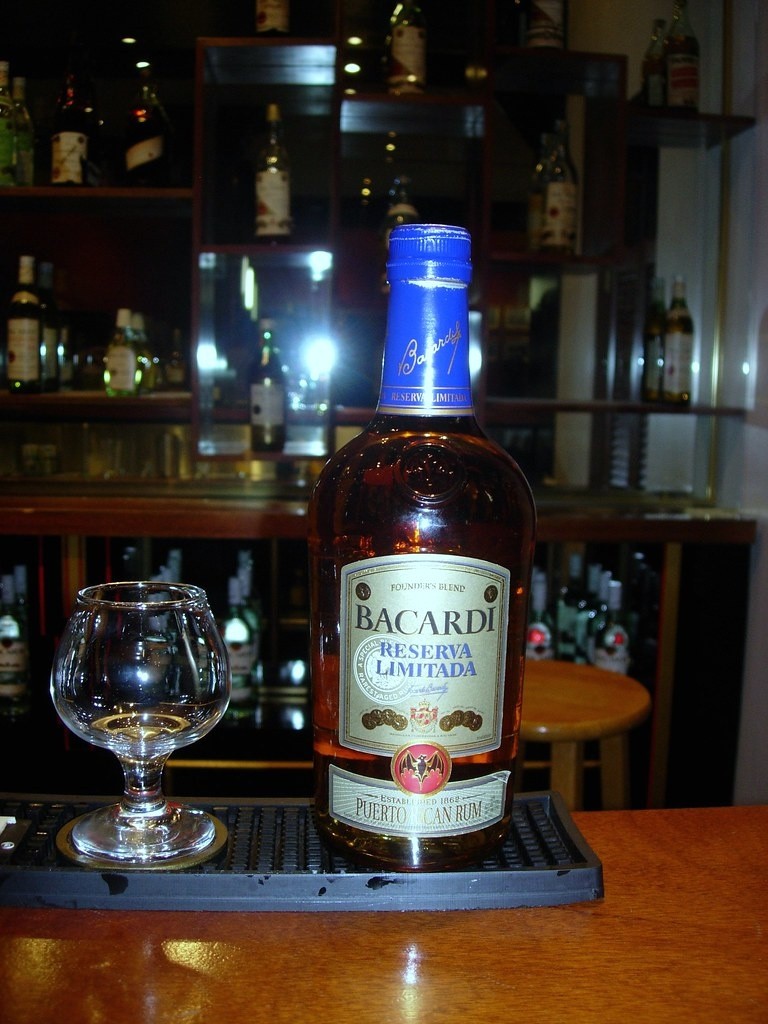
[307, 220, 541, 871]
[0, 1, 715, 717]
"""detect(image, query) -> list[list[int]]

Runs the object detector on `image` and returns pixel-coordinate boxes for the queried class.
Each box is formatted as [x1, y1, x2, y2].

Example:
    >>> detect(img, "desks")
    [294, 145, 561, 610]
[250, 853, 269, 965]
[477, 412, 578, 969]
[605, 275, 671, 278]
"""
[0, 787, 768, 1024]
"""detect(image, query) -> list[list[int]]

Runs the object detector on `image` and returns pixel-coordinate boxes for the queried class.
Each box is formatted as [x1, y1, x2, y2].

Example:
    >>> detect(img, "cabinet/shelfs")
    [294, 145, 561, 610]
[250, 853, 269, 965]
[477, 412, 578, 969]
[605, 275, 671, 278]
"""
[1, 35, 757, 512]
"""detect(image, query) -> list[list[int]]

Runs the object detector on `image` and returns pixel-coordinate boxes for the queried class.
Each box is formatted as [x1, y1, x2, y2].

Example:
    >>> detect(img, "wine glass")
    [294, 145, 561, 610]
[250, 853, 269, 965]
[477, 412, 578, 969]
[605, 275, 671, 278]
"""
[48, 584, 230, 867]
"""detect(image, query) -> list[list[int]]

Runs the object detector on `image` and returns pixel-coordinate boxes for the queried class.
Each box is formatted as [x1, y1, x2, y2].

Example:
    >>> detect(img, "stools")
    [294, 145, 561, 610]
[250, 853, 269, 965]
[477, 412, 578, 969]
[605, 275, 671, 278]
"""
[516, 657, 651, 812]
